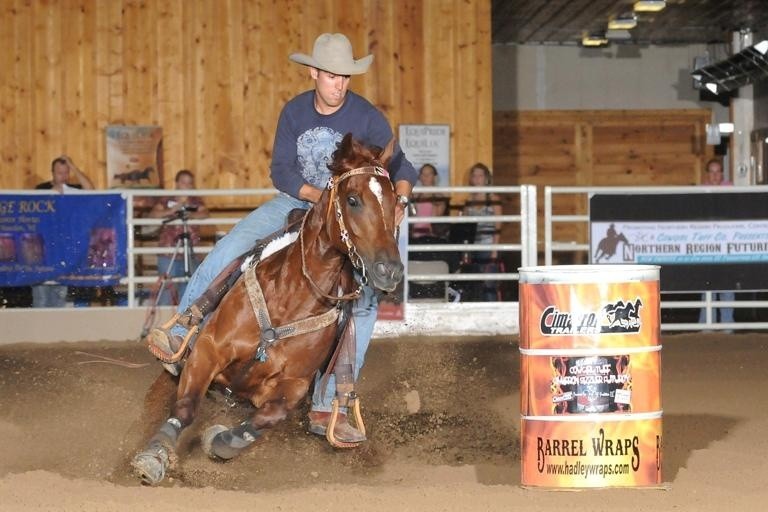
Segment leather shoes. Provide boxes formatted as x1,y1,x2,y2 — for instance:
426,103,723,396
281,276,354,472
152,327,189,377
309,411,366,443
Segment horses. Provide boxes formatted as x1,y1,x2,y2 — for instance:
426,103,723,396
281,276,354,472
129,129,406,491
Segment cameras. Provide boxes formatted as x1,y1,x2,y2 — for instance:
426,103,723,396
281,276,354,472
181,202,198,212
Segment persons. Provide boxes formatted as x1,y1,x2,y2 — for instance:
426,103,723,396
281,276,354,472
133,168,215,305
150,32,422,443
697,159,740,336
406,161,449,239
30,155,96,308
405,215,459,300
459,160,506,301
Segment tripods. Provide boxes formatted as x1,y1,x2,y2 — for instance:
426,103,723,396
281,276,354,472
141,232,195,340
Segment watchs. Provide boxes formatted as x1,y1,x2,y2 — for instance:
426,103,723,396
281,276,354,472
395,193,409,209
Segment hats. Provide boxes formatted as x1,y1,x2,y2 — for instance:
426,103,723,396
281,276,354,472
289,34,375,74
